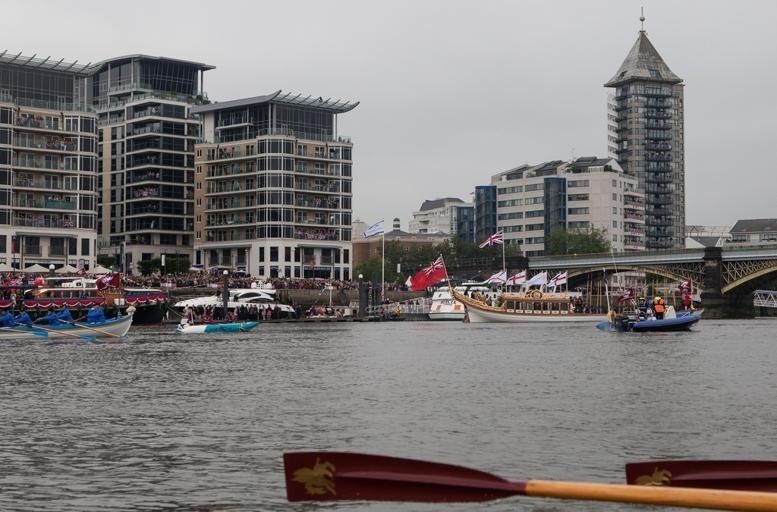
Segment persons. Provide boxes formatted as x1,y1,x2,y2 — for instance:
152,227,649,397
651,294,665,320
568,296,596,314
464,286,502,308
1,272,404,299
182,300,401,324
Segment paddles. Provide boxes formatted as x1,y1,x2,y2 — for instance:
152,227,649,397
624,461,777,494
282,451,777,512
13,322,96,341
0,328,49,337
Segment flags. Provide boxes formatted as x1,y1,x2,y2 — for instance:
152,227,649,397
410,257,448,292
479,231,503,249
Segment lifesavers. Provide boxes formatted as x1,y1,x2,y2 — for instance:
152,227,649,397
532,289,543,299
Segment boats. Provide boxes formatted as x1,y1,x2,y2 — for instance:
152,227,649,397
4,288,135,340
176,309,260,336
595,249,710,336
447,229,650,325
427,282,494,322
